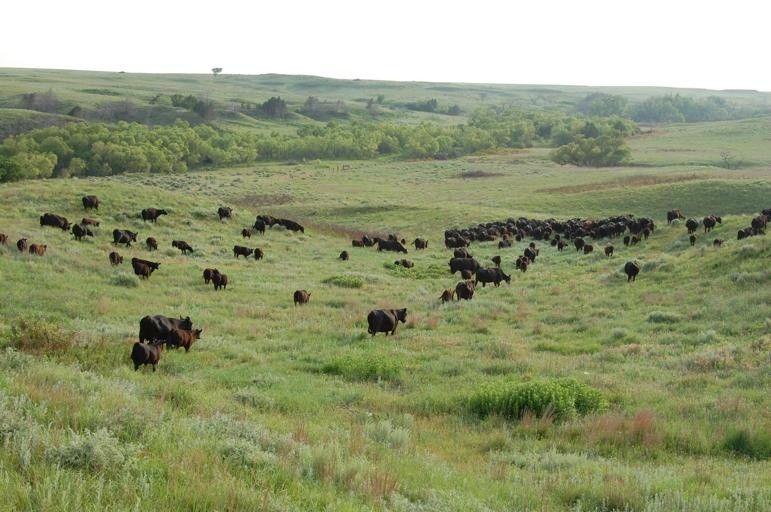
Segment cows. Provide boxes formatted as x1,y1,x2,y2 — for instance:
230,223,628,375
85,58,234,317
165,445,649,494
440,289,454,303
0,231,47,257
666,209,724,247
40,196,195,281
241,214,306,239
442,214,655,283
350,231,429,255
394,258,414,269
217,206,233,220
340,251,349,261
130,314,202,373
367,307,407,337
231,245,264,260
294,289,311,306
202,267,229,291
454,280,476,300
736,209,771,240
